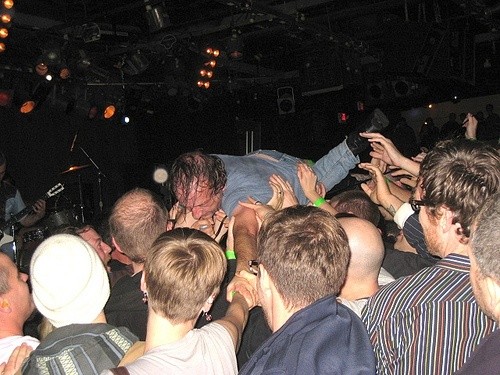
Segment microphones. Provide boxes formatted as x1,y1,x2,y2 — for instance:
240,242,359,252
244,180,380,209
70,132,78,152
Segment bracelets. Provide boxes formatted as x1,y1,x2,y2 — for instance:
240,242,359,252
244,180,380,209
225,249,236,260
313,197,324,207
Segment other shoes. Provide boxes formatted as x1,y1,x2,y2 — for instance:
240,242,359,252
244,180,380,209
347,110,390,153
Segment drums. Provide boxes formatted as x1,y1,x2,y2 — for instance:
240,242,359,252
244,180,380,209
24,225,51,245
43,206,78,231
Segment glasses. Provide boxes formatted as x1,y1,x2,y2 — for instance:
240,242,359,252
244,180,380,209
408,196,443,211
247,259,263,275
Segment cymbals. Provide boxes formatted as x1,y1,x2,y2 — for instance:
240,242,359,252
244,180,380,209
58,163,93,175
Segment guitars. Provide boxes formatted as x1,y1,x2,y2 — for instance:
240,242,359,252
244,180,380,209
0,182,65,247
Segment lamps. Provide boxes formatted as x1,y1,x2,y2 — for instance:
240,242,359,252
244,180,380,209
0,0,500,122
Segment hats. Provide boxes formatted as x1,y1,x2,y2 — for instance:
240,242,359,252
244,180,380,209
27,233,111,327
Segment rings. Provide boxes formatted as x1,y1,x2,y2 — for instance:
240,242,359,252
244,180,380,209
199,225,207,229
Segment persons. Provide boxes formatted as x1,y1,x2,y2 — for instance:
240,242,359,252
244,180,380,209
0,110,500,374
